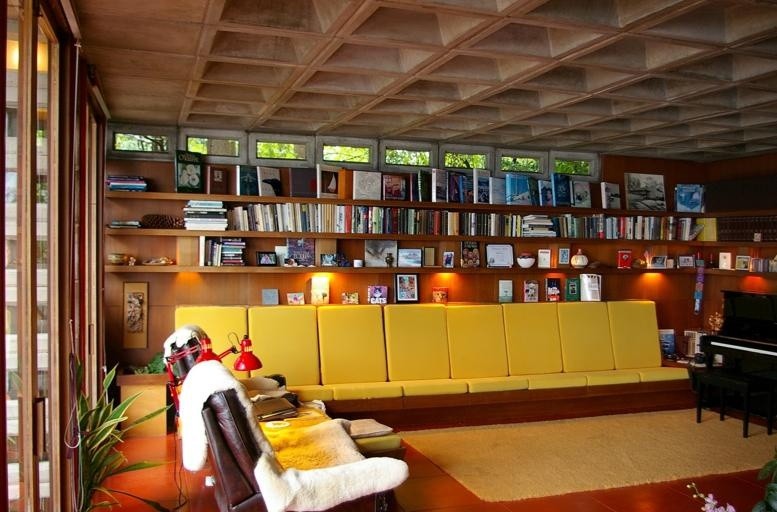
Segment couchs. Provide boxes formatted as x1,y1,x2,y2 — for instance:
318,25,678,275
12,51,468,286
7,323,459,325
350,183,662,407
158,300,696,432
164,321,406,512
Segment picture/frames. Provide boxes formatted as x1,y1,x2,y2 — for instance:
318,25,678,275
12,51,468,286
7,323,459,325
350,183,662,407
121,282,149,349
316,163,341,199
677,254,695,269
394,273,420,303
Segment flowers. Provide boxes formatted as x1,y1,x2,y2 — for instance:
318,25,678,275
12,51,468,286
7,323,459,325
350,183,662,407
687,482,737,512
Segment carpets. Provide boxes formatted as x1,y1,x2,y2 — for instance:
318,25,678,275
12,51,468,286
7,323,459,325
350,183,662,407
393,404,776,502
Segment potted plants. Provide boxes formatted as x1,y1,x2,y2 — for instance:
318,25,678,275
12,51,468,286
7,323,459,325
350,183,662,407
115,352,170,436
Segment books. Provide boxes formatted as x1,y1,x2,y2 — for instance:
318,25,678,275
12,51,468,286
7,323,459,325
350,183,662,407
107,220,142,229
262,277,387,304
275,238,337,267
289,164,591,208
254,396,298,422
433,287,449,304
183,200,717,241
175,151,282,197
718,251,777,272
499,274,602,302
365,239,480,269
105,175,148,192
601,173,705,213
176,235,246,266
717,216,777,242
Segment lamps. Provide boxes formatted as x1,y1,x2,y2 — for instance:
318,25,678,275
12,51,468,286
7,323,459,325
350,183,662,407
165,333,262,443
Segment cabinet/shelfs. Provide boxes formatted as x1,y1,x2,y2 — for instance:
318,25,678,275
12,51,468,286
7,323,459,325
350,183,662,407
99,180,704,276
703,196,777,287
